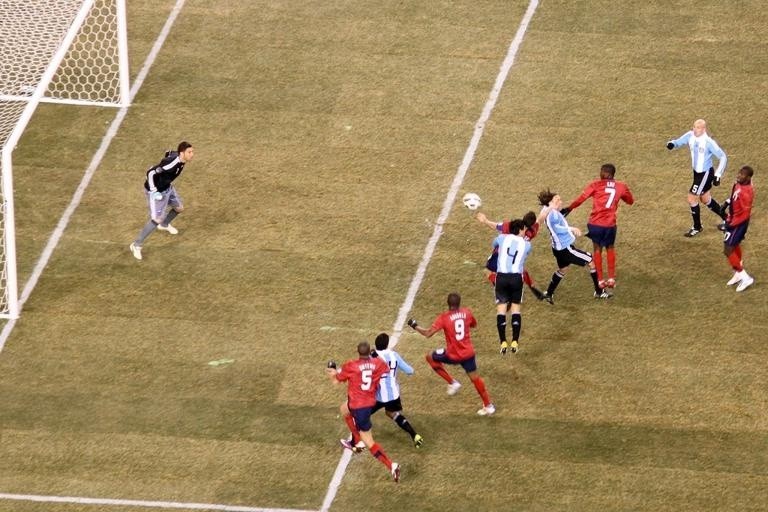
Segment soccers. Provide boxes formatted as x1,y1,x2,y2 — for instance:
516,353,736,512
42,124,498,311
463,193,481,210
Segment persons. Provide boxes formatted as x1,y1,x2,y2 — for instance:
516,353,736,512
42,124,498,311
493,219,533,354
560,163,636,290
476,201,558,303
127,140,195,260
665,119,731,236
326,341,401,482
719,166,755,291
340,333,423,454
406,293,496,416
536,187,613,304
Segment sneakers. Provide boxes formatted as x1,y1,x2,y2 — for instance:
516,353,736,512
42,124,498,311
157,224,178,235
339,428,425,482
498,340,522,357
685,222,724,237
727,269,754,292
542,279,616,304
447,379,495,417
130,243,142,259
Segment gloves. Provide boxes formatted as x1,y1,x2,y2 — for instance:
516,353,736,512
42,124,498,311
560,207,570,218
407,318,418,329
667,143,722,188
327,345,381,368
